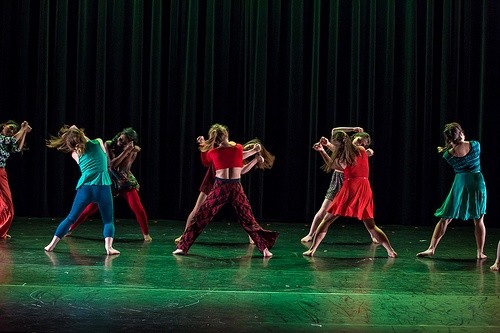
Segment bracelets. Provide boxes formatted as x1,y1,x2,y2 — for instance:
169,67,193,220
254,147,257,153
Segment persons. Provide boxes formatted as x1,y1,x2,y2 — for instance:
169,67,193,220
63,127,153,242
174,138,275,243
171,124,280,257
0,121,32,239
416,122,488,258
489,241,500,271
302,132,397,258
301,127,380,243
43,125,120,254
